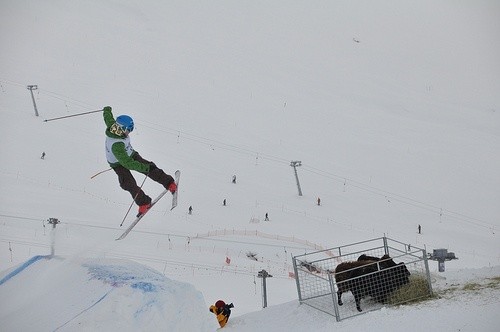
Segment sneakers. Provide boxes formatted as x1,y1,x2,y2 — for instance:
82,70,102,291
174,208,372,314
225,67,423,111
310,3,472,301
167,178,177,194
138,199,151,213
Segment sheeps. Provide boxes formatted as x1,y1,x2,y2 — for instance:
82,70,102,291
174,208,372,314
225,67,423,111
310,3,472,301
335,254,413,312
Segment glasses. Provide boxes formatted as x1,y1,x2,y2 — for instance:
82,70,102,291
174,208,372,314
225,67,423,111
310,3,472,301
128,126,133,131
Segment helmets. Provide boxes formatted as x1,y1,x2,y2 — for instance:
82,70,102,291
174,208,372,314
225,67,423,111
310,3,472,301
116,115,135,133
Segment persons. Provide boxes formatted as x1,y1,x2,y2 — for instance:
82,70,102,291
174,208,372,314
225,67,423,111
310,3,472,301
103,106,176,216
209,299,231,328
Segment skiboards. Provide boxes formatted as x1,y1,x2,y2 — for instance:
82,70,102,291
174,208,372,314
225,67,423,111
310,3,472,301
114,170,181,241
